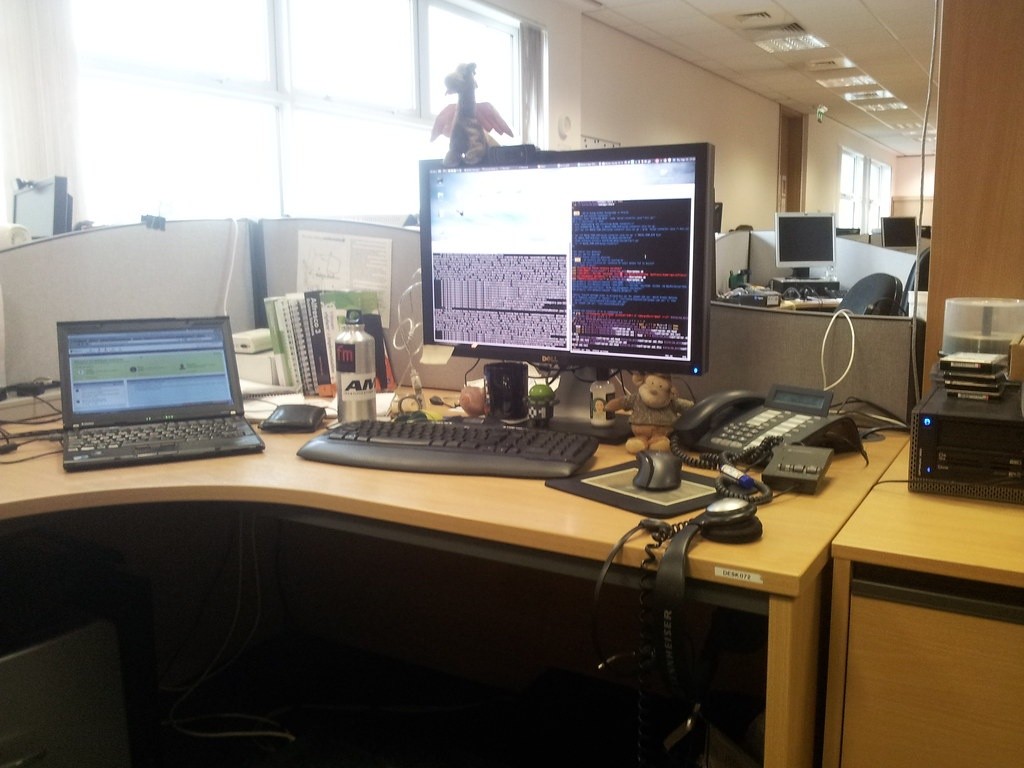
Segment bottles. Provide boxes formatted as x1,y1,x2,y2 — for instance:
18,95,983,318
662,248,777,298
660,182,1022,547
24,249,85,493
336,309,376,423
590,367,615,428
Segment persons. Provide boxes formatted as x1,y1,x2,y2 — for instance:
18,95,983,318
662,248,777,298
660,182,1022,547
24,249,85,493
729,225,753,231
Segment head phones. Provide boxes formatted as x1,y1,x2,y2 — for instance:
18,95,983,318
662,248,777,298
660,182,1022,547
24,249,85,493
651,496,763,707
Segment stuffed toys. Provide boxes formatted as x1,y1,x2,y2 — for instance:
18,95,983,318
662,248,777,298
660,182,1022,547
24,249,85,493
604,370,695,455
430,61,514,166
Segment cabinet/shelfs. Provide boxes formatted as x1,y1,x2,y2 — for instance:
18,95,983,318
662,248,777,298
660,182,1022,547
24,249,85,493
825,437,1024,768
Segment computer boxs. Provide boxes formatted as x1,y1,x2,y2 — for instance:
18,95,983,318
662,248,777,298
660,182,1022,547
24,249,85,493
768,278,840,299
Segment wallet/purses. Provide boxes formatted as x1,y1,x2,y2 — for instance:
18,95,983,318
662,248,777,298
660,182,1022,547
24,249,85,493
258,404,326,434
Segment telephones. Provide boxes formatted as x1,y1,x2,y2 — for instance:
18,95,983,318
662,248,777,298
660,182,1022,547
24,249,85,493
675,385,862,467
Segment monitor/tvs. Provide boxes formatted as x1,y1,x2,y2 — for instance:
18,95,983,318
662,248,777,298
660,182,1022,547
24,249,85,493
13,175,73,240
419,143,717,445
881,217,918,254
775,213,836,280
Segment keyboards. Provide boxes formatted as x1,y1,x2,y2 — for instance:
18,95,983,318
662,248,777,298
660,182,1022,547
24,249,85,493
296,420,600,479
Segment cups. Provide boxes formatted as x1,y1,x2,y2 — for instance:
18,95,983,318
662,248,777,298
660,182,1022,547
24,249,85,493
826,270,837,281
484,363,529,422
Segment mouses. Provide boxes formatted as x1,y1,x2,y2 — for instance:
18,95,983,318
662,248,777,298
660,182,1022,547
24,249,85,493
633,449,683,490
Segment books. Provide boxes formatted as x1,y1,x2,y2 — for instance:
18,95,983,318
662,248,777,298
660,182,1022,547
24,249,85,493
264,288,396,395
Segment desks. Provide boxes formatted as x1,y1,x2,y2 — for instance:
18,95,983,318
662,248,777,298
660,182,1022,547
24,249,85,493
1,224,913,767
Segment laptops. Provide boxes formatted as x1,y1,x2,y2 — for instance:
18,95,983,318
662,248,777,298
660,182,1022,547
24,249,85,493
56,316,266,472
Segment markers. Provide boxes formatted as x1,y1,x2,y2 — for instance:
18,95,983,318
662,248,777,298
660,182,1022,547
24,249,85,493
722,464,754,487
